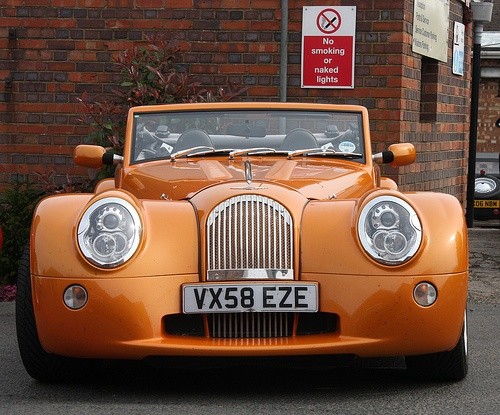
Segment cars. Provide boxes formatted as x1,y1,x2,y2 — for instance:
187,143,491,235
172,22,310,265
473,152,500,222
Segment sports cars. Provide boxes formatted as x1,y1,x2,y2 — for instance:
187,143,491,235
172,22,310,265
13,101,471,385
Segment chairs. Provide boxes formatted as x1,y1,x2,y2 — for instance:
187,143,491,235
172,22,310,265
173,128,212,155
281,128,319,150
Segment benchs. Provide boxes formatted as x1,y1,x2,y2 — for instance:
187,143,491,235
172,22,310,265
152,133,333,150
